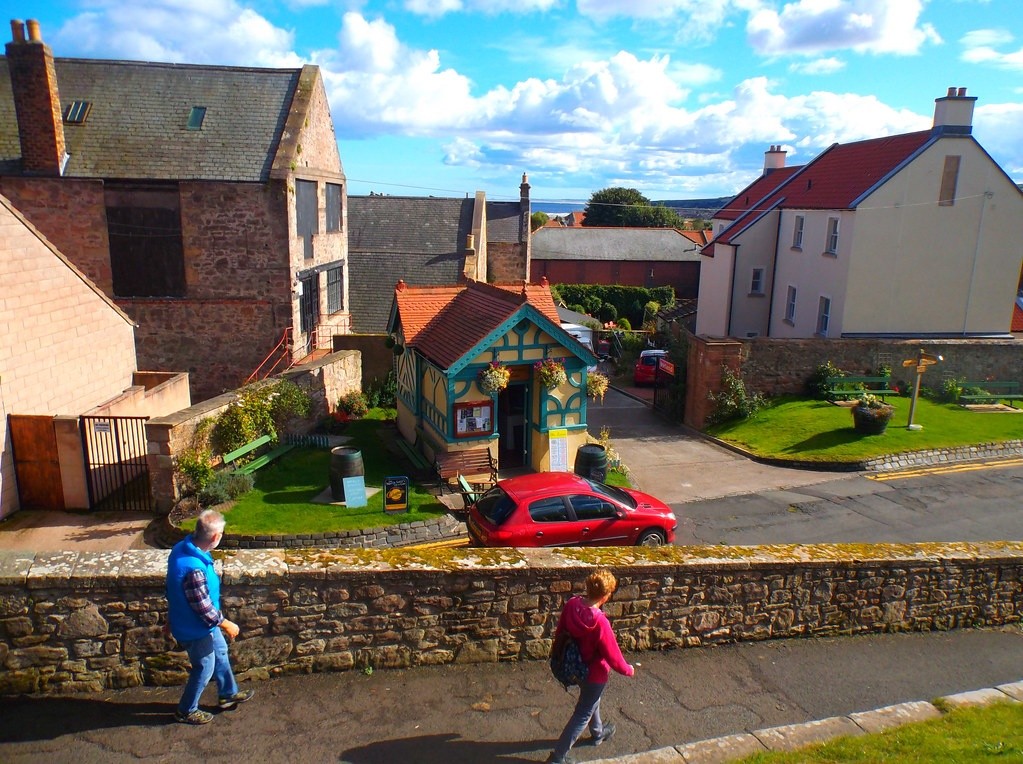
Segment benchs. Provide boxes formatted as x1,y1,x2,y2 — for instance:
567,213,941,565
955,381,1023,407
825,378,898,401
436,449,499,499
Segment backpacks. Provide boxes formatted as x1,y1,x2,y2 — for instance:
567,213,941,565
551,623,599,687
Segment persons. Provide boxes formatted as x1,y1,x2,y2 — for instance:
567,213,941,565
546,569,635,764
165,510,254,724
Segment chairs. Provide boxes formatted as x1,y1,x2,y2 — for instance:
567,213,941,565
456,469,495,520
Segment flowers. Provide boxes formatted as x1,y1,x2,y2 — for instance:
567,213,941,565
533,355,568,389
479,360,510,391
585,370,609,405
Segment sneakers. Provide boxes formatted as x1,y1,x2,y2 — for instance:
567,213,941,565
218,689,255,708
175,709,213,724
591,723,616,746
545,751,575,764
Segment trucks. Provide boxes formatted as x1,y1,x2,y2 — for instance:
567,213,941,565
561,322,595,354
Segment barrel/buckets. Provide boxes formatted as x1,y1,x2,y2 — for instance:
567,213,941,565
329,446,365,501
574,443,607,484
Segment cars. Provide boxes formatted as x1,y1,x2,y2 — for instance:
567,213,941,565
634,349,674,387
465,471,679,548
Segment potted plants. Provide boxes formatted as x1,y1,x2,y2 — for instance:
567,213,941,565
850,392,896,445
702,365,772,429
